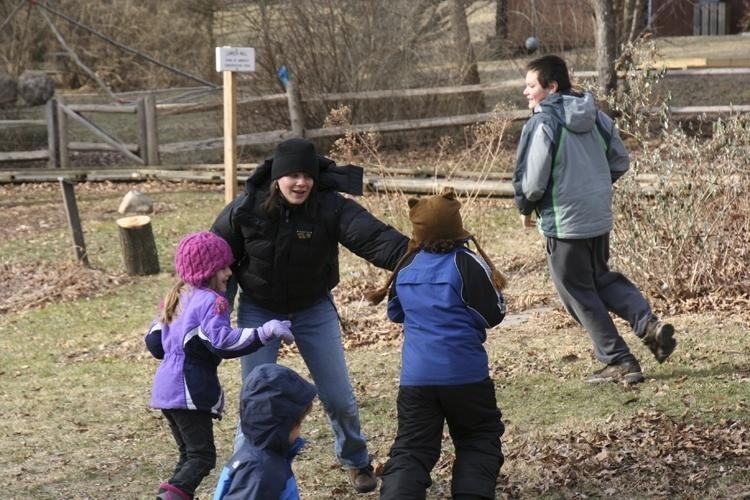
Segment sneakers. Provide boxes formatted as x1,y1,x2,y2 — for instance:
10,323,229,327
349,465,377,493
650,323,677,364
584,359,644,384
156,482,193,500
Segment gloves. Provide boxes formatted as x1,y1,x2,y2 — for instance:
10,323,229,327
256,320,295,345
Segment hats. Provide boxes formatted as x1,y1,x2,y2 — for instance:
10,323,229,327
159,232,233,314
271,139,319,182
368,187,507,305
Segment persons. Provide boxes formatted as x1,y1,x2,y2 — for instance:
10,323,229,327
145,230,294,499
511,54,677,385
211,362,319,499
207,136,412,494
368,190,508,500
